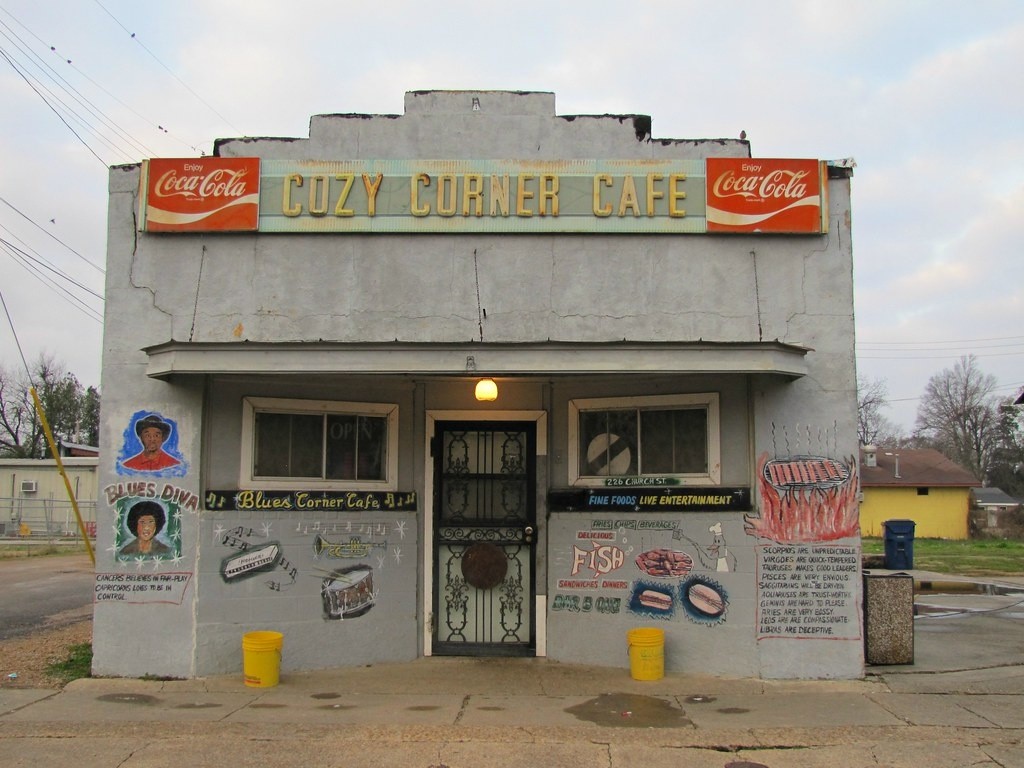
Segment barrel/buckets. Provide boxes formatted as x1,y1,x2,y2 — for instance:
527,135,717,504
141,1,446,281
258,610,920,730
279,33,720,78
242,631,284,688
626,627,665,680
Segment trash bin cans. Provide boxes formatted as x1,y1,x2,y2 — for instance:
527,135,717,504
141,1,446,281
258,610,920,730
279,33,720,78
881,518,917,571
862,570,914,666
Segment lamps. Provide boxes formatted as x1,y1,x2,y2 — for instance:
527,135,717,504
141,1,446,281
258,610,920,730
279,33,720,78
474,378,498,402
471,96,481,112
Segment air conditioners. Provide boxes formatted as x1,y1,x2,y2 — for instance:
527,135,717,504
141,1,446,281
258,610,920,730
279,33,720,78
20,480,36,492
